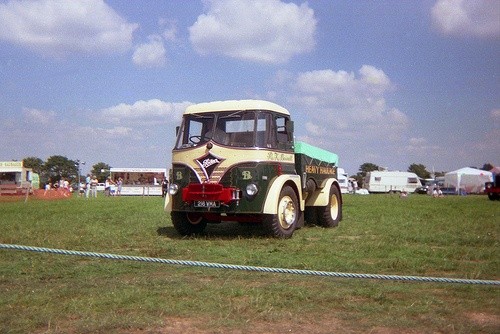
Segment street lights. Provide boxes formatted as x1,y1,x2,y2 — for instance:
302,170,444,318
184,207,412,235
74,159,86,192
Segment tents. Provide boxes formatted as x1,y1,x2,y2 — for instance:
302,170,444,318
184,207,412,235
444,166,494,195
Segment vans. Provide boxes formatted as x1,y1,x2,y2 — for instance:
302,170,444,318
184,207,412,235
416,180,456,194
364,170,422,193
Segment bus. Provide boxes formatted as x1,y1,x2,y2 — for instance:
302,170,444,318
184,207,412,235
163,99,343,239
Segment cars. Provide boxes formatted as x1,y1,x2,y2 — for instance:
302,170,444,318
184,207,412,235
80,182,86,188
96,183,105,190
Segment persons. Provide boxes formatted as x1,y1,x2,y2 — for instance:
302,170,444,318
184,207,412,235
161,177,168,197
59,177,69,189
348,180,358,194
84,174,98,198
117,176,123,195
105,177,113,190
